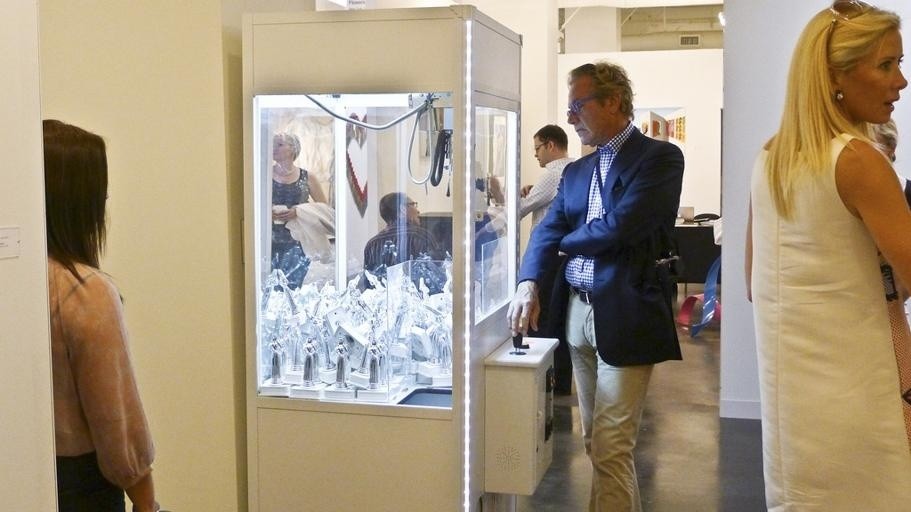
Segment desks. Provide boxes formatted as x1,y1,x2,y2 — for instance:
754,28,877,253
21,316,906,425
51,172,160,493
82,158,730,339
671,218,722,295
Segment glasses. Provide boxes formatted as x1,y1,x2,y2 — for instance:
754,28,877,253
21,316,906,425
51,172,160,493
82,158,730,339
407,202,417,207
535,142,550,154
567,95,599,118
828,0,871,38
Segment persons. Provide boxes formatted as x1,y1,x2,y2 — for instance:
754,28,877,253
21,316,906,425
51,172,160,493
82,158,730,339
508,63,685,510
859,110,911,300
363,190,446,292
272,133,325,286
490,124,582,394
42,116,164,512
746,3,911,510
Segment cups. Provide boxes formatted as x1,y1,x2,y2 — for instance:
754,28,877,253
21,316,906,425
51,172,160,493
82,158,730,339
272,206,288,225
682,206,695,222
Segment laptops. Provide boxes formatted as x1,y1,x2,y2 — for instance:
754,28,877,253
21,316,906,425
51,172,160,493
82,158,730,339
677,206,694,222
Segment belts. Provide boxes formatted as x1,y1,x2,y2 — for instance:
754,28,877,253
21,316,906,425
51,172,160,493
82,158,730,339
575,288,592,306
